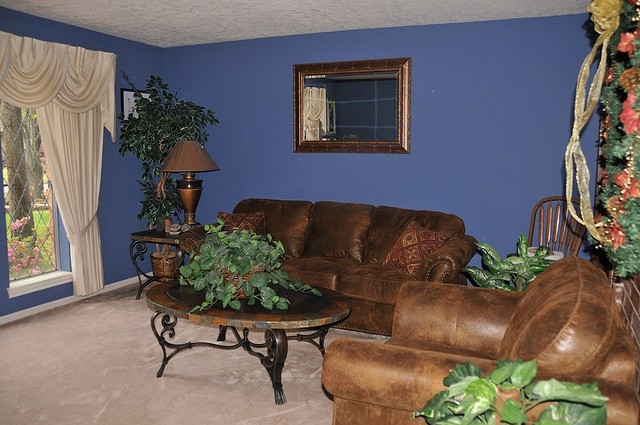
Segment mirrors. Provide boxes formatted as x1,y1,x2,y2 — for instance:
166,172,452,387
293,56,412,154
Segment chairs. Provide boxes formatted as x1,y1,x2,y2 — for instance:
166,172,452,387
523,195,586,256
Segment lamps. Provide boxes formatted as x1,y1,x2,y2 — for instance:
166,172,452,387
160,140,220,228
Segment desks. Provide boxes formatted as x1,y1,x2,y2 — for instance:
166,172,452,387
129,229,186,300
145,280,352,405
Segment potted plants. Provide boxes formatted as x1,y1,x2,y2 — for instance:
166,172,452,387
116,69,220,283
178,216,322,313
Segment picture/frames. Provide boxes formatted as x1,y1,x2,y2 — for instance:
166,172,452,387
325,99,337,136
120,87,152,124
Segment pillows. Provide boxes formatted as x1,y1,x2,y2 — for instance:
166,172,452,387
382,219,452,277
256,214,311,259
217,211,265,235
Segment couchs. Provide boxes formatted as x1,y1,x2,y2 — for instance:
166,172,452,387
321,254,640,425
179,198,478,336
